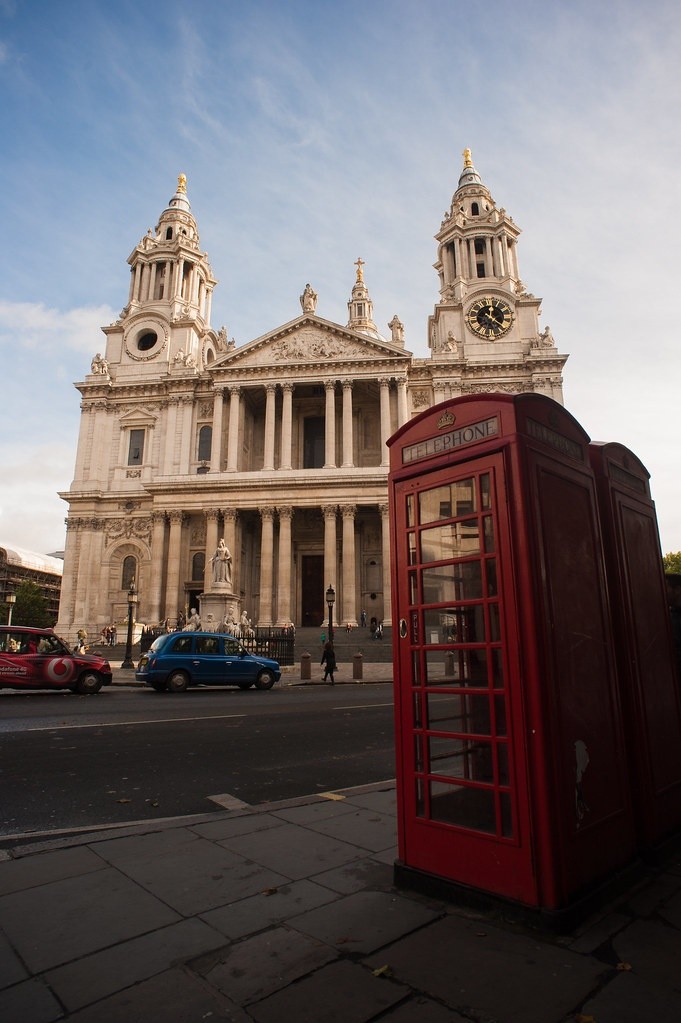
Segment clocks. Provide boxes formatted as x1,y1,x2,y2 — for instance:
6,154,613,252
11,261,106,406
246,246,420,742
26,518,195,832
464,296,515,341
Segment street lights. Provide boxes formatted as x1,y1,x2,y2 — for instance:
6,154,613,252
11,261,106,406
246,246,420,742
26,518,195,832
5,587,18,652
323,584,339,672
120,586,138,669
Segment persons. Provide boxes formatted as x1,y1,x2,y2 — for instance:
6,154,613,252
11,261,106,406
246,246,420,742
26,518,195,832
320,642,335,686
347,609,384,641
388,314,404,342
444,637,457,673
321,631,326,645
302,284,317,311
208,538,232,583
180,302,191,320
223,606,243,637
182,608,201,632
100,624,117,647
442,330,457,353
282,623,295,647
174,348,191,366
240,610,256,643
161,611,183,632
218,326,228,345
537,326,555,347
90,353,109,374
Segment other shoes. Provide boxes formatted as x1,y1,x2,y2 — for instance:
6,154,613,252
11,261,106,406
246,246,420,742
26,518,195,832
330,682,334,685
322,678,326,681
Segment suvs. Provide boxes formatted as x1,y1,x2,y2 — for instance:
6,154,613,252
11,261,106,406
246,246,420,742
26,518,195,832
134,630,282,694
0,623,114,695
